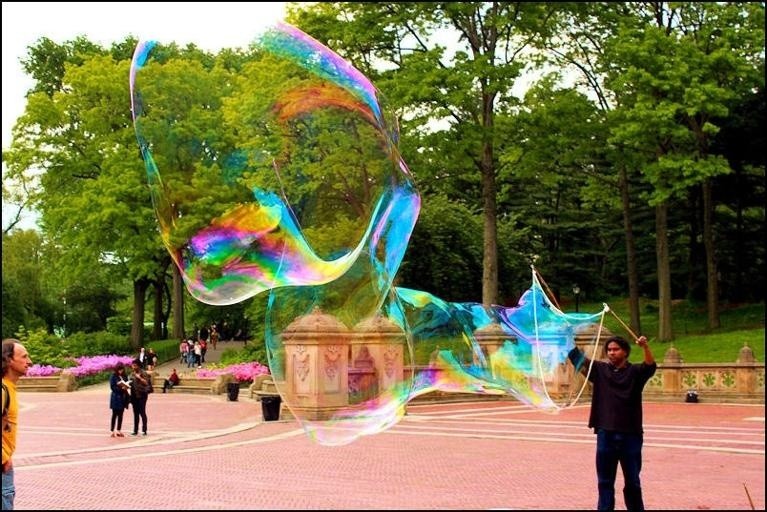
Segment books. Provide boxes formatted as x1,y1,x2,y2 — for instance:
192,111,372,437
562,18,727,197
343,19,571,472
116,379,131,391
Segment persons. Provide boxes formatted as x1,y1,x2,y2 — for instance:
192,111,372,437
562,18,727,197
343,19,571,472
110,361,130,437
192,322,198,342
199,324,209,345
160,368,178,394
210,323,221,350
147,348,159,372
197,336,209,362
130,359,149,435
566,323,657,511
179,339,189,364
138,348,148,370
0,339,32,510
193,342,203,368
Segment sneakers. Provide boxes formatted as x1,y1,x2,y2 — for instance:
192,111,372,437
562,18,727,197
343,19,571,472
131,432,137,436
110,433,118,438
117,432,125,438
140,431,147,437
